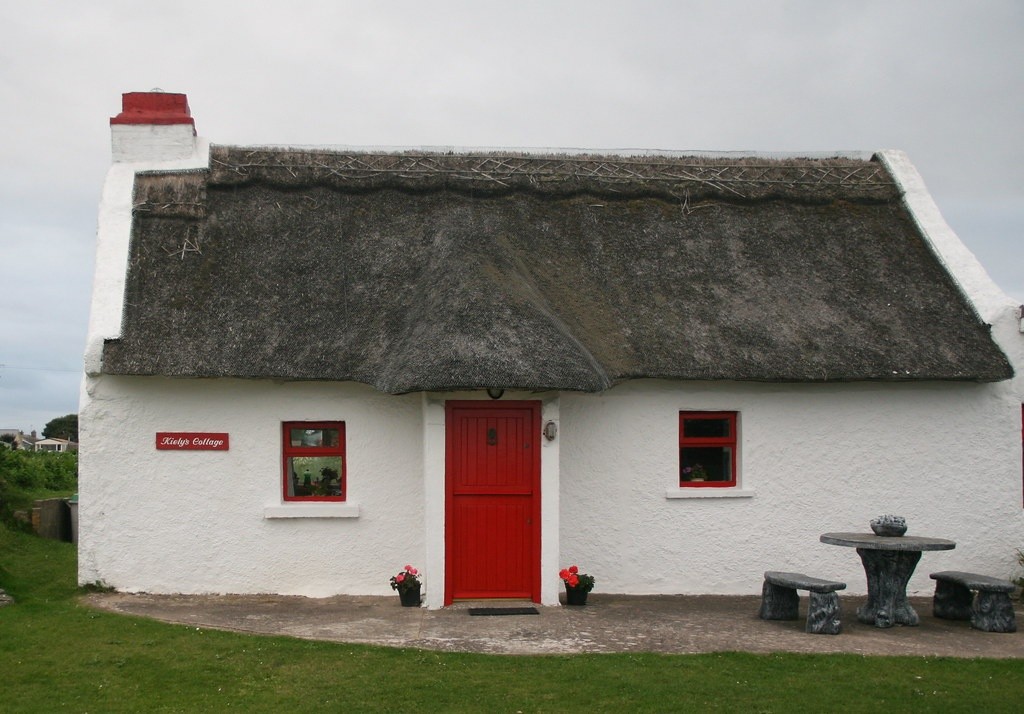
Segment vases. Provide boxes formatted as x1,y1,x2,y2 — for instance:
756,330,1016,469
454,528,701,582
566,587,588,606
691,478,704,481
399,581,420,607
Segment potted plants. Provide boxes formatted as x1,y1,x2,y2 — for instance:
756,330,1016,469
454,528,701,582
871,514,907,537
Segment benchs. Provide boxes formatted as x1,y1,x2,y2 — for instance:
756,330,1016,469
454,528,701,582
758,571,846,635
930,571,1017,633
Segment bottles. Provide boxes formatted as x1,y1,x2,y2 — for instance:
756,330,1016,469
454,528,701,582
304,469,311,488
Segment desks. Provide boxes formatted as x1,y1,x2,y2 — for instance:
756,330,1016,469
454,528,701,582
820,532,956,628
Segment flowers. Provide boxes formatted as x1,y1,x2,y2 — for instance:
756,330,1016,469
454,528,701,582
390,565,423,594
559,565,595,592
682,463,705,478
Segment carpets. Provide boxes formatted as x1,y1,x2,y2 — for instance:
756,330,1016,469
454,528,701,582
468,608,540,616
